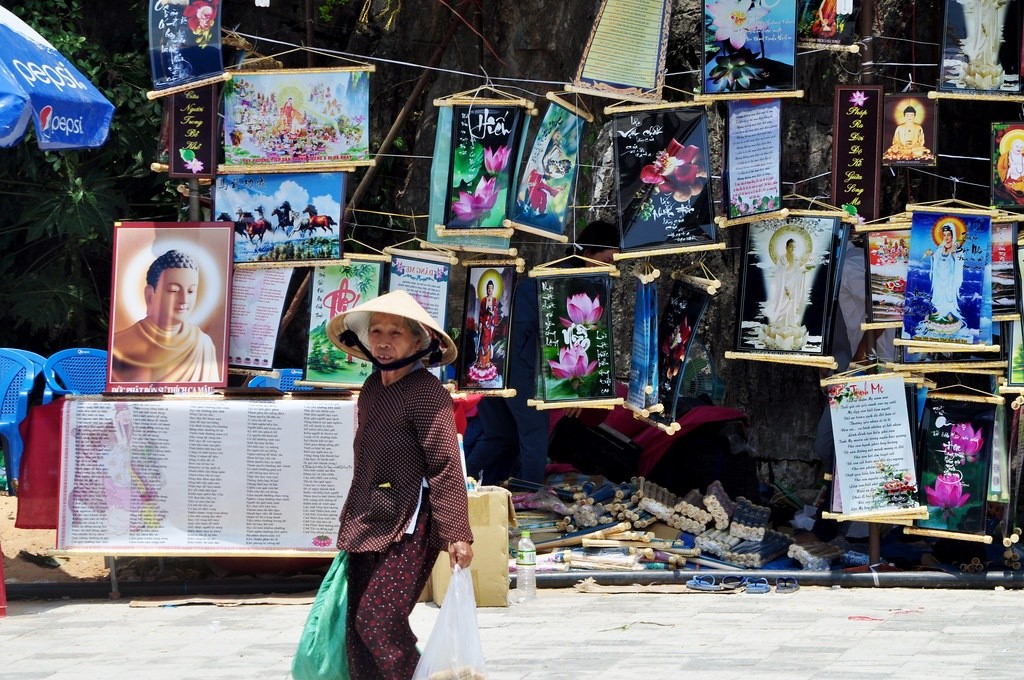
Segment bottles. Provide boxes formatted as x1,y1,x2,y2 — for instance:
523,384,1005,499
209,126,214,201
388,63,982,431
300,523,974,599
516,532,537,603
841,550,895,567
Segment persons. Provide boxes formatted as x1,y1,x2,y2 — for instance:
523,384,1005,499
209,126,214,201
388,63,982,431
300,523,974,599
327,290,473,680
814,237,926,545
462,219,623,491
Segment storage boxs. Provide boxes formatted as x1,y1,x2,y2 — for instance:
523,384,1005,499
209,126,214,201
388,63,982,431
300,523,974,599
429,485,519,608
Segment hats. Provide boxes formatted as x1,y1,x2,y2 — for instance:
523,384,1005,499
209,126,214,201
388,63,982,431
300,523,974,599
327,290,457,368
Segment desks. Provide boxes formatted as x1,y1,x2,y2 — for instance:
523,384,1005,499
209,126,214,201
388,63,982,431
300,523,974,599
57,386,467,600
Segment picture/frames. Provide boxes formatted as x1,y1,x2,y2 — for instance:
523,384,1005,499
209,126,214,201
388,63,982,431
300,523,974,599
106,221,235,394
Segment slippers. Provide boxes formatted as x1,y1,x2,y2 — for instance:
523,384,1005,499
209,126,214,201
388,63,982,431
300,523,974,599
775,576,800,594
720,576,747,589
745,578,772,593
686,576,723,590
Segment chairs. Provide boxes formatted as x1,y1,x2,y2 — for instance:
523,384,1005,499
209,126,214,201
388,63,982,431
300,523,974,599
0,348,110,499
248,368,346,392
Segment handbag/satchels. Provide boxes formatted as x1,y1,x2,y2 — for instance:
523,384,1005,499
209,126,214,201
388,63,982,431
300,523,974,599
290,549,351,680
411,562,491,680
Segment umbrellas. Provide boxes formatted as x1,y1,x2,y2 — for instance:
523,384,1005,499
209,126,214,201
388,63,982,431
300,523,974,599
0,4,117,152
